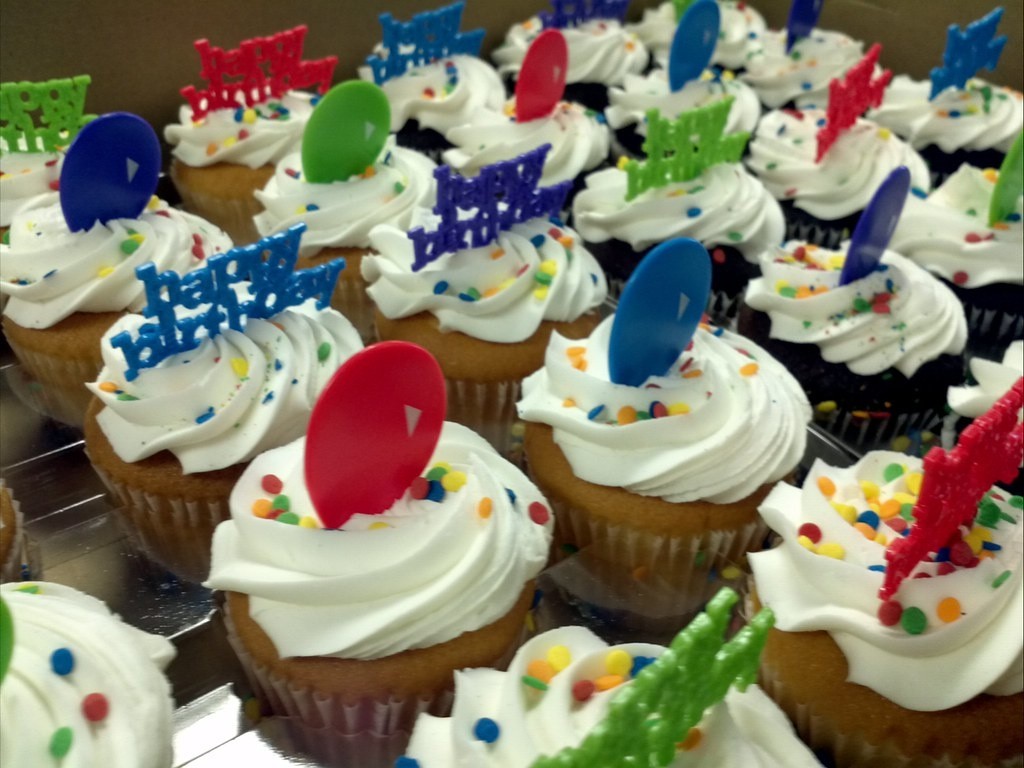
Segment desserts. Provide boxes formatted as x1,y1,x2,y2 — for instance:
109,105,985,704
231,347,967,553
0,0,1024,768
